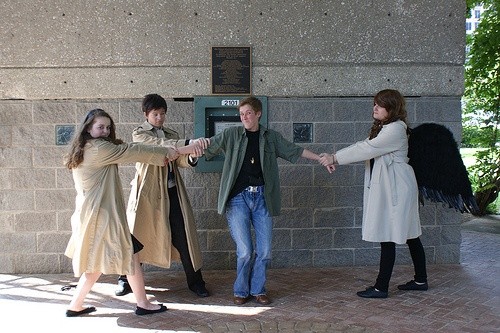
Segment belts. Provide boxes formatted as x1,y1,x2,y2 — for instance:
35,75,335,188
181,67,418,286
244,186,260,192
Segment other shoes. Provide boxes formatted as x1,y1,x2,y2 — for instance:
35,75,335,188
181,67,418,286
234,297,247,304
255,295,270,305
135,304,167,315
115,284,131,296
188,286,209,297
357,286,388,298
66,306,95,317
397,280,428,291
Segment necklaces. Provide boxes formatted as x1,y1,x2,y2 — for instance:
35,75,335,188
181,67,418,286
248,147,258,164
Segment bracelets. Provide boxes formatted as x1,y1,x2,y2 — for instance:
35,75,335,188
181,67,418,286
332,154,336,165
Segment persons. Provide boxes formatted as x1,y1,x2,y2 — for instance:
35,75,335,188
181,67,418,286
190,96,335,305
116,93,210,297
64,109,202,317
318,89,428,298
